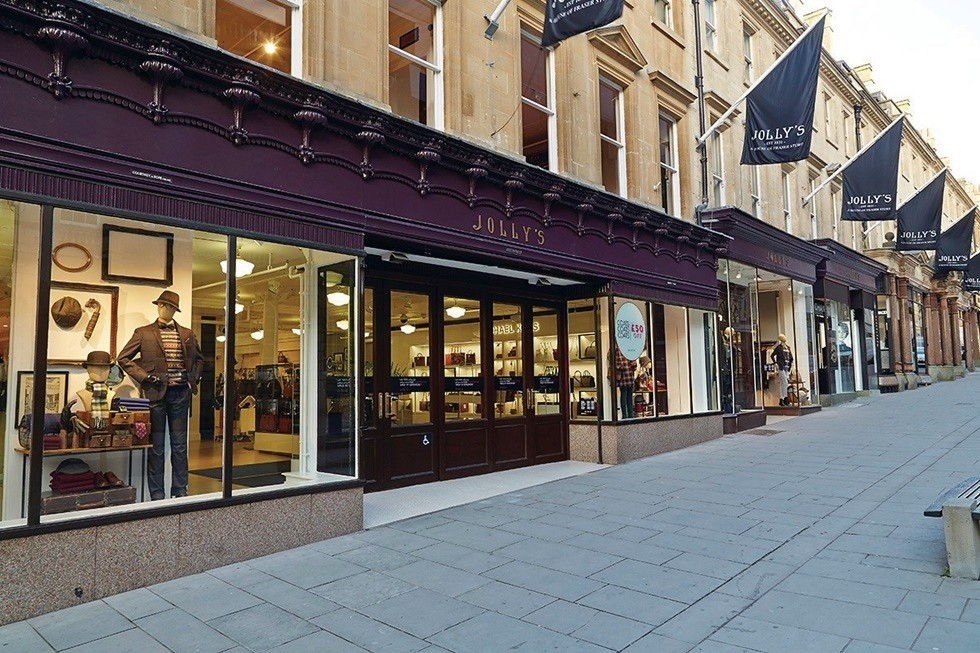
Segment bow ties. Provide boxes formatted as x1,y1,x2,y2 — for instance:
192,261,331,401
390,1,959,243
158,323,175,330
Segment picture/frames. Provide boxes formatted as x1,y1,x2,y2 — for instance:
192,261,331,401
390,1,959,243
15,371,69,429
101,223,173,286
47,282,119,365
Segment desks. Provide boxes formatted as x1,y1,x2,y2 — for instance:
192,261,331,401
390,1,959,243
14,445,154,518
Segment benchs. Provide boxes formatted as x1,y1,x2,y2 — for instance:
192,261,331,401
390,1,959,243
923,477,980,580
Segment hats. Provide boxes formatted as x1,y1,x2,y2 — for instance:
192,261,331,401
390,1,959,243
55,457,90,474
82,351,114,368
639,356,651,367
152,291,181,312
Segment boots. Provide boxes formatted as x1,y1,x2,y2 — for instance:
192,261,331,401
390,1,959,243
784,397,790,406
779,398,786,406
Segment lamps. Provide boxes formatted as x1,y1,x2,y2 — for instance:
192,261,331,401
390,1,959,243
446,299,466,318
336,315,348,330
220,252,255,278
400,319,416,334
223,299,244,314
327,286,350,306
250,329,264,340
291,325,304,335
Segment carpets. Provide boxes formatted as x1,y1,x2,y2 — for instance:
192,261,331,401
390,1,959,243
189,460,291,488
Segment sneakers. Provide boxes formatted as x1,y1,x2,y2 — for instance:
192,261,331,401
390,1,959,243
92,470,109,487
104,471,122,487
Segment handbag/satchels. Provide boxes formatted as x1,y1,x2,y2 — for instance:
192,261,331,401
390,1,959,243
496,347,516,358
535,342,558,361
510,409,518,414
633,371,653,416
537,366,559,385
445,346,476,365
585,340,597,358
445,392,480,412
419,393,431,411
581,397,598,414
497,402,504,413
573,370,595,387
414,353,426,366
496,369,503,376
509,361,515,377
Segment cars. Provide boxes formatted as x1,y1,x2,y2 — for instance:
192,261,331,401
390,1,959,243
823,338,875,369
915,337,926,365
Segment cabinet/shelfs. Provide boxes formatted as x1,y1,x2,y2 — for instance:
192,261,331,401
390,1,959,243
410,333,668,418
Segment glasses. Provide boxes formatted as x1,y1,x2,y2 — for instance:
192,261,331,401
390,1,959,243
158,302,174,308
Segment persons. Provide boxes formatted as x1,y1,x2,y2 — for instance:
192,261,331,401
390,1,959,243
118,291,204,502
639,356,652,369
771,334,793,406
77,351,116,411
723,327,736,402
838,322,851,351
616,347,637,419
